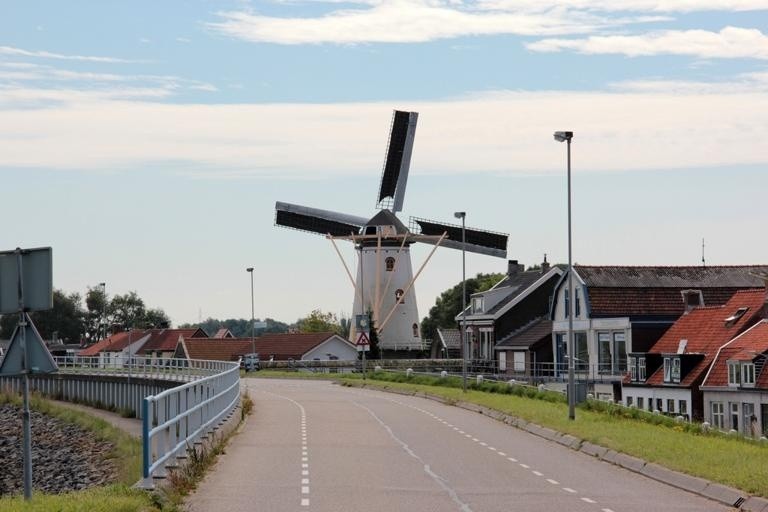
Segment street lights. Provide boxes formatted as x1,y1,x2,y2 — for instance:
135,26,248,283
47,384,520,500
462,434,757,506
100,282,107,356
453,210,468,393
245,266,256,352
553,129,578,419
